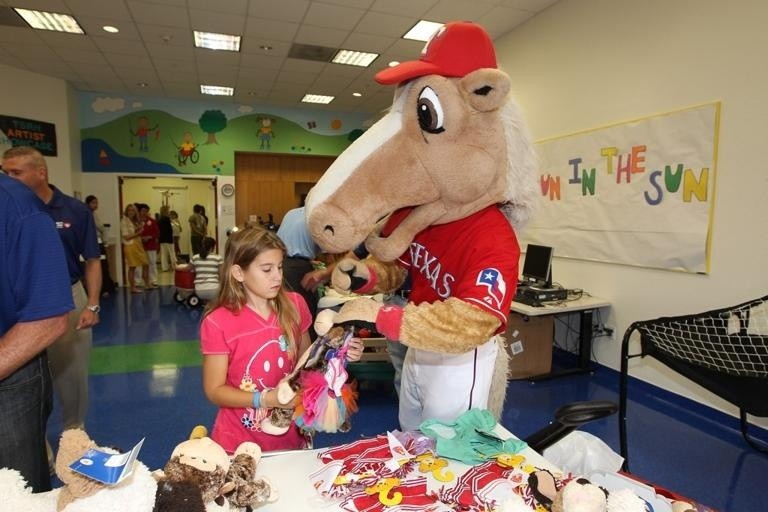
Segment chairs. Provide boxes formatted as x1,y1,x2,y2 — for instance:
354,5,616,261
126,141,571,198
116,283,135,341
620,295,768,474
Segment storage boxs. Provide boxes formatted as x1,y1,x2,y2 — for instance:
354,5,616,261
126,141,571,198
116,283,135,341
506,313,556,381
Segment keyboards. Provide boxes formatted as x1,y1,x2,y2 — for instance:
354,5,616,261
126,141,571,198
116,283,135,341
512,291,535,306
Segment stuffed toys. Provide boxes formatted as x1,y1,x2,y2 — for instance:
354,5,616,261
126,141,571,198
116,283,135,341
303,20,542,431
0,428,157,512
151,425,271,511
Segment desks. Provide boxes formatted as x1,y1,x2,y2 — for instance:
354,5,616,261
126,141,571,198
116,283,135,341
510,296,611,387
33,421,566,512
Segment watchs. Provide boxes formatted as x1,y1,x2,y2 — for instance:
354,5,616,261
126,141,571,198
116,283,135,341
86,304,100,312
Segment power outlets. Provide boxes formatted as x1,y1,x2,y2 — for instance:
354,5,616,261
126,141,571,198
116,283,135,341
605,325,616,340
593,323,603,336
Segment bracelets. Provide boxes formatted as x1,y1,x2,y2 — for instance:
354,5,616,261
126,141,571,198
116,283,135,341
253,391,260,407
260,387,276,409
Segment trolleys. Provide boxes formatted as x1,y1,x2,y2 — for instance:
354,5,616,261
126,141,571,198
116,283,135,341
172,262,202,307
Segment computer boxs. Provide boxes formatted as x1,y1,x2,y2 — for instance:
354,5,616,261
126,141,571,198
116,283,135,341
523,289,568,301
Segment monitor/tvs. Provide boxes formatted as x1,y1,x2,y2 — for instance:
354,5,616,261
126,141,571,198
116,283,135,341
521,243,560,291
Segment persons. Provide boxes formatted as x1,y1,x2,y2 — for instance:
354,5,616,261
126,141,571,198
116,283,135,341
276,206,320,342
188,237,224,299
200,225,363,454
188,204,208,255
2,173,78,494
86,195,181,294
1,148,103,474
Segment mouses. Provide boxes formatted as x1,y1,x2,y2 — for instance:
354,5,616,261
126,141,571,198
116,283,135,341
531,301,543,307
